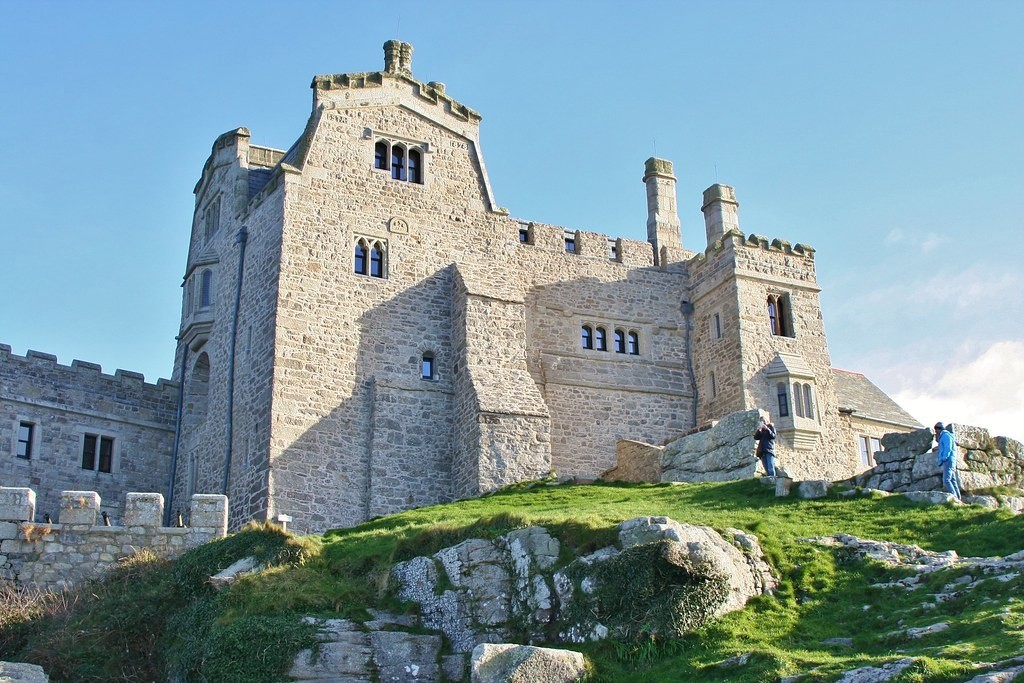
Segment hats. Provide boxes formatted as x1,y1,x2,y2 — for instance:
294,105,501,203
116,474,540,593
934,422,944,430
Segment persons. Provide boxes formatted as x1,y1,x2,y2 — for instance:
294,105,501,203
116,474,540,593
754,416,777,476
934,422,961,500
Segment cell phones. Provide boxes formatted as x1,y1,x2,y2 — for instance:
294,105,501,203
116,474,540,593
759,421,764,426
